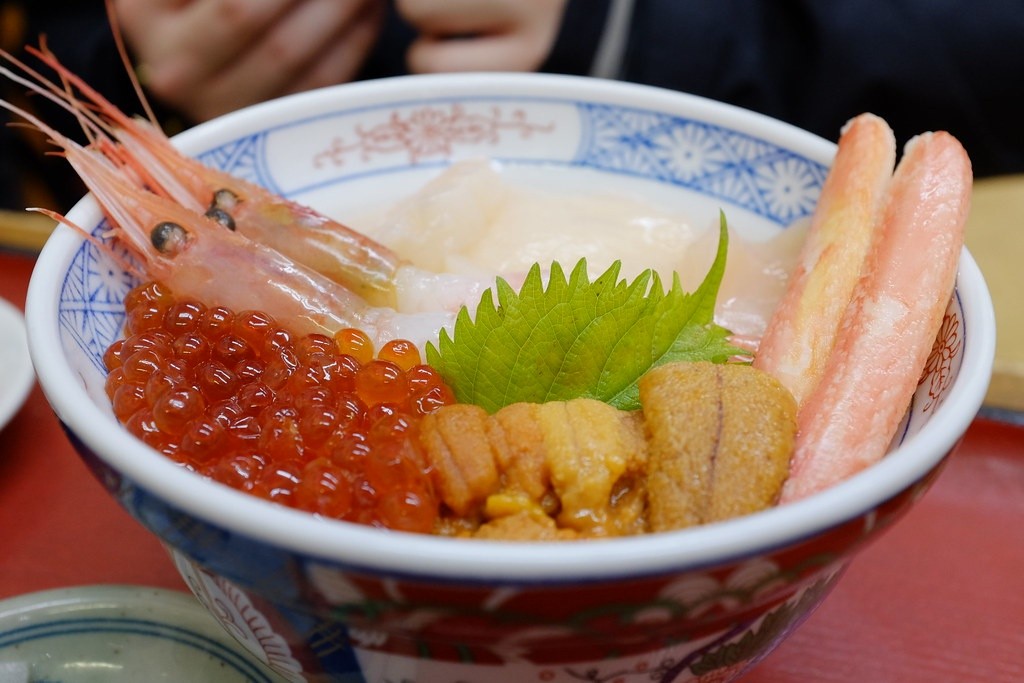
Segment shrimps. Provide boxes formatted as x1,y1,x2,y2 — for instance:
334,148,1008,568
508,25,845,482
0,0,806,365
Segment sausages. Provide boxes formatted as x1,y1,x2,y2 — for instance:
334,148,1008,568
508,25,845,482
754,114,973,508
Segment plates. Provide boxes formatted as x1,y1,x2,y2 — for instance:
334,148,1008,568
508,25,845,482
0,583,277,683
0,293,36,428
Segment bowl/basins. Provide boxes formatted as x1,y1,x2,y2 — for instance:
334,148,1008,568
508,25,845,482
28,72,994,683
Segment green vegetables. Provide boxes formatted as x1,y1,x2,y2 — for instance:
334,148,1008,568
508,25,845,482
424,207,756,408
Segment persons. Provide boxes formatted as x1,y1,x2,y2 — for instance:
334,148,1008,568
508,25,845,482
0,0,1024,183
